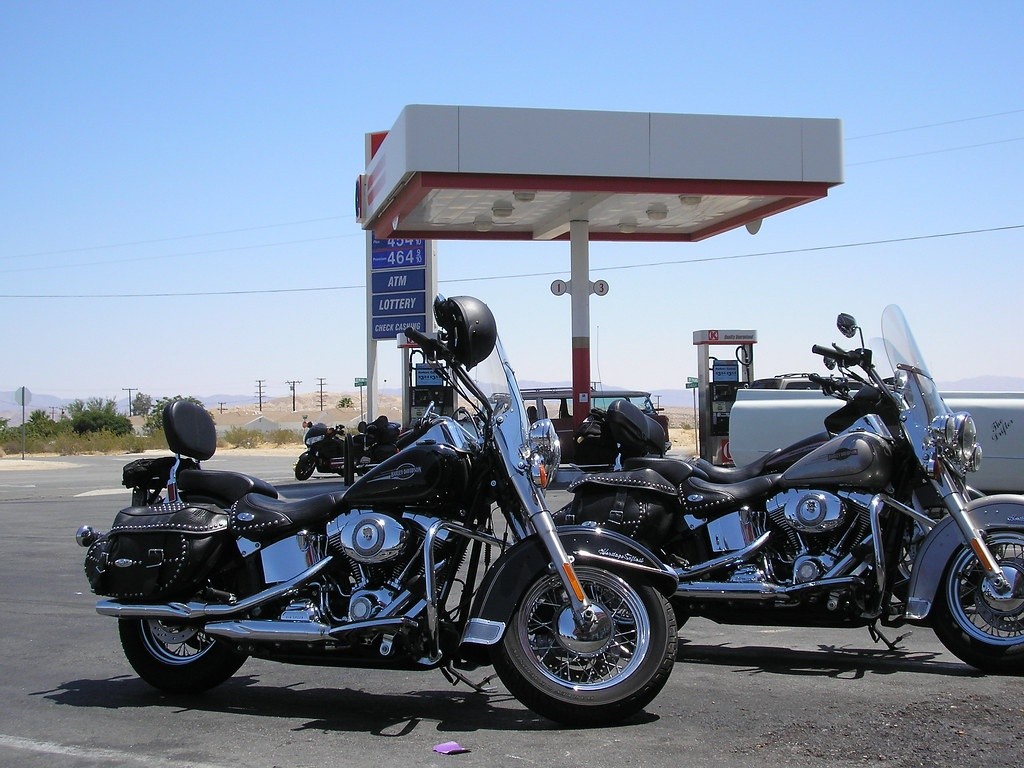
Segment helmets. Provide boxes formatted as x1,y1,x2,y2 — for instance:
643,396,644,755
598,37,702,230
824,382,906,448
440,296,497,372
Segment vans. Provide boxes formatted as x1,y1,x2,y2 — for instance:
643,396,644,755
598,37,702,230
480,387,672,466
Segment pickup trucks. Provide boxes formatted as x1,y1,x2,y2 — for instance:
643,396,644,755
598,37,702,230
728,388,1024,612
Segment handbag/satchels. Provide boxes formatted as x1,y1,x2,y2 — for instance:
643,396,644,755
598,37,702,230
573,408,616,465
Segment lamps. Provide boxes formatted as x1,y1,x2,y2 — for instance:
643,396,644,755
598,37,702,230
473,215,494,232
618,221,641,233
646,209,670,221
492,202,515,217
678,194,702,206
515,192,536,202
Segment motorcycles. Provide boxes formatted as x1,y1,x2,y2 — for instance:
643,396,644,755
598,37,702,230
74,296,679,730
294,415,426,480
546,304,1024,676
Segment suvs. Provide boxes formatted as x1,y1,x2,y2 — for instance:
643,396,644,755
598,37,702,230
748,372,867,393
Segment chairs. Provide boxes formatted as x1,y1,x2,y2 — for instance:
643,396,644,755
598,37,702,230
526,405,537,418
